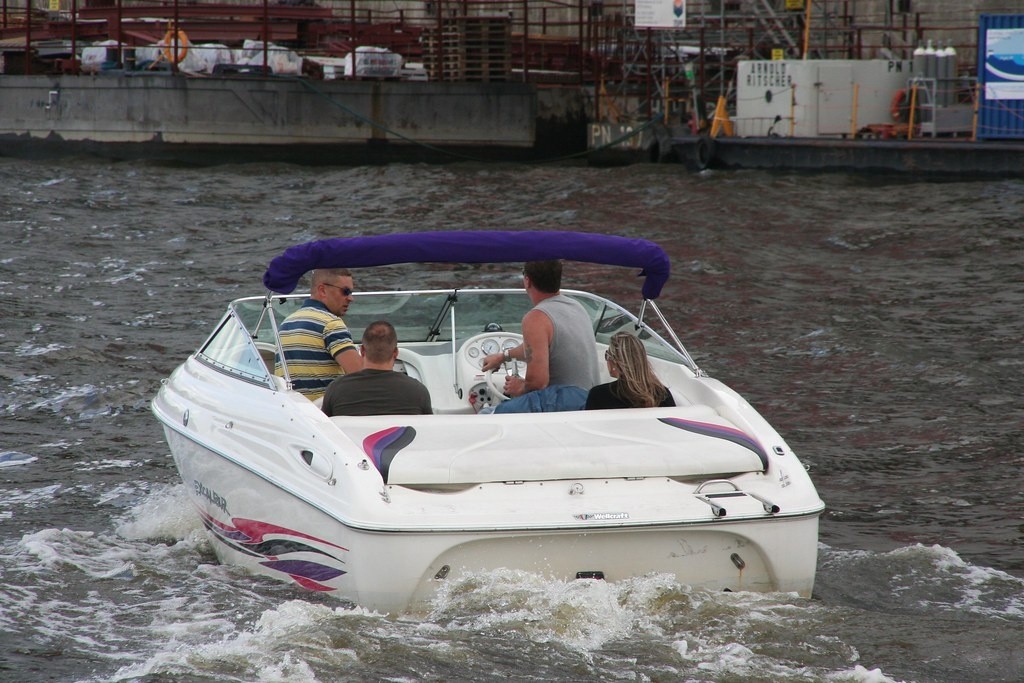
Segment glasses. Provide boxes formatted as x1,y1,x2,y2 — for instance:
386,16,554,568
320,282,353,297
603,349,615,365
521,268,526,277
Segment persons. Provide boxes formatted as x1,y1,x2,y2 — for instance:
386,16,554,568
481,259,601,399
274,269,364,403
585,330,677,410
320,319,434,416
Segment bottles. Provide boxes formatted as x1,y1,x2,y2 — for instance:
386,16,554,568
511,357,520,399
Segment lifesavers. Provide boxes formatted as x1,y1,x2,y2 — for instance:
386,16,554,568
893,88,907,119
166,27,187,62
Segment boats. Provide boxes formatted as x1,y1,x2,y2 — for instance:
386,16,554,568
149,230,829,623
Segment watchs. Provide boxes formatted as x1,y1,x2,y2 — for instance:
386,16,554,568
503,348,512,362
